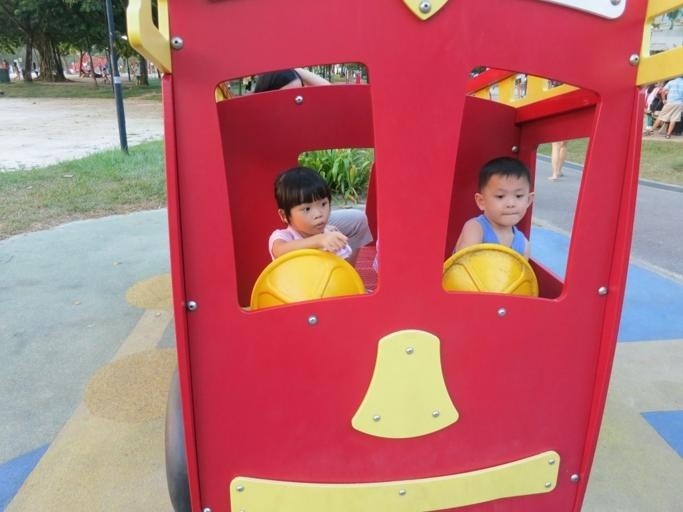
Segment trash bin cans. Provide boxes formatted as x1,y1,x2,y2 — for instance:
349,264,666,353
0,69,9,82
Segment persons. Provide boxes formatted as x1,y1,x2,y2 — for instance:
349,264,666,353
1,60,8,70
549,141,567,181
451,157,536,263
267,166,353,262
253,68,374,267
642,77,682,139
32,63,38,77
101,68,110,85
11,62,20,81
130,63,141,86
